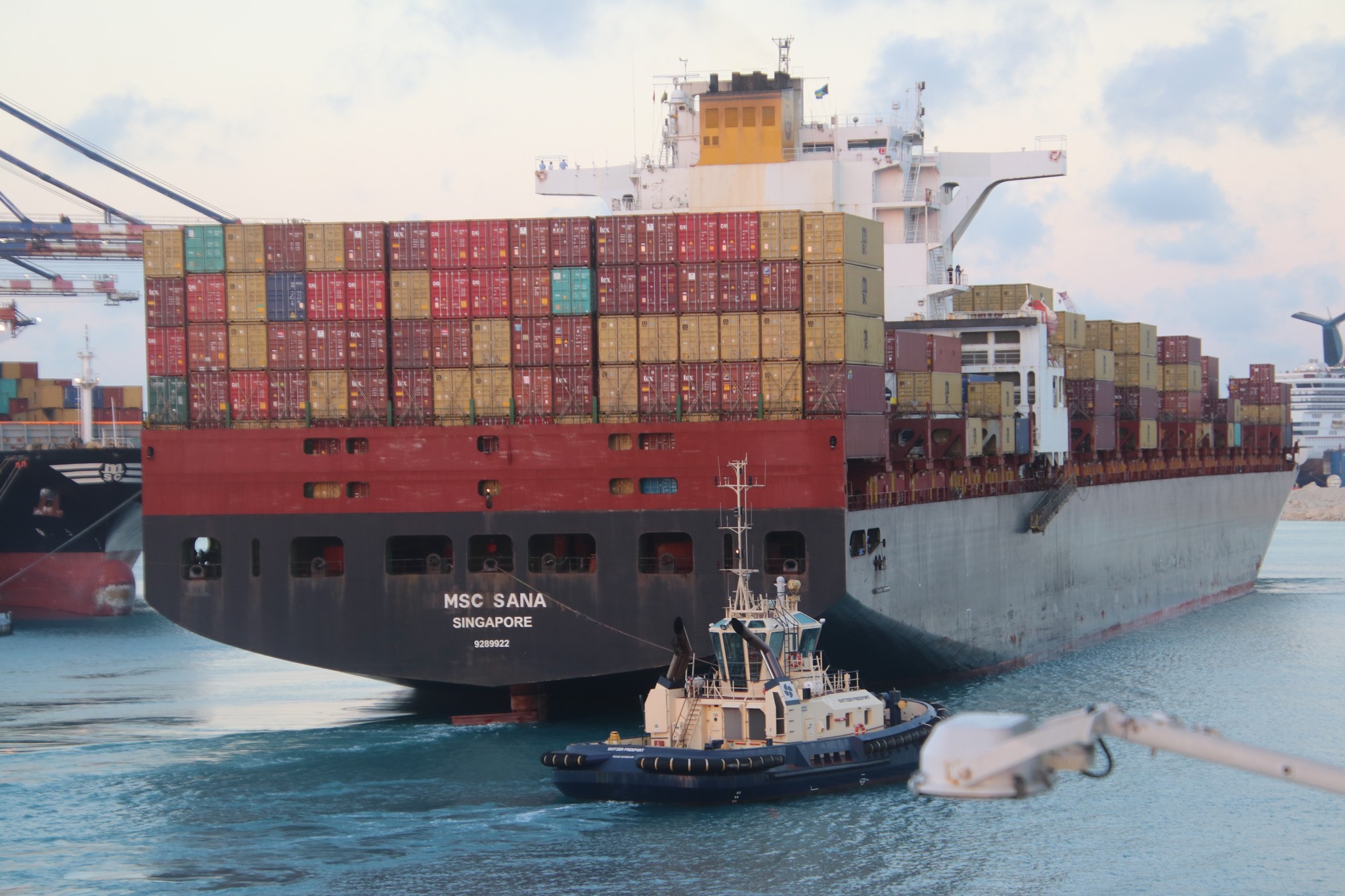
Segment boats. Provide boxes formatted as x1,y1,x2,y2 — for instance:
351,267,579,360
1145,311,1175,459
538,456,949,809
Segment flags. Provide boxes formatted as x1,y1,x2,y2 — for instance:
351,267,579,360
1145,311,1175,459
815,83,829,99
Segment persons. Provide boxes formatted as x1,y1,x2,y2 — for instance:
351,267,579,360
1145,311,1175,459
539,159,568,171
946,265,963,284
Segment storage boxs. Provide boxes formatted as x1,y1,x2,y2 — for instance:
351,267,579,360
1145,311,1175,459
0,212,1295,515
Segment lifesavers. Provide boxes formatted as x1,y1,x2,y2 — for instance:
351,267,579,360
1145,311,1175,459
788,651,801,667
687,676,693,690
538,171,546,180
855,724,865,736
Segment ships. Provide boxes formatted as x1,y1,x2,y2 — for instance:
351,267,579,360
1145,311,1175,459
1,100,245,624
1273,308,1345,488
137,28,1303,703
